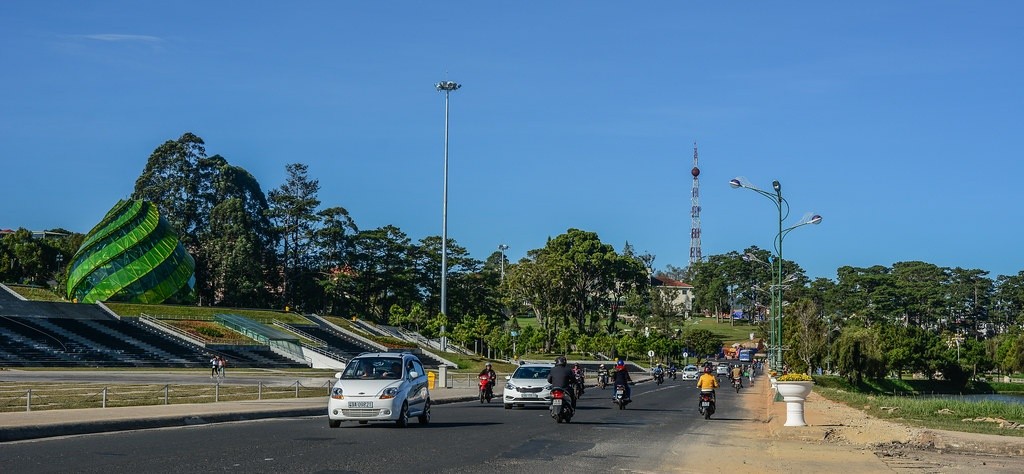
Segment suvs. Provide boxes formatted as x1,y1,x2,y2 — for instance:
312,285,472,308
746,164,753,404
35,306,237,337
328,352,431,428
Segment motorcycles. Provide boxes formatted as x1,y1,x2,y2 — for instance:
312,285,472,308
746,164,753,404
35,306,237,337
571,372,584,400
653,369,676,385
597,371,632,410
728,368,755,393
477,374,495,404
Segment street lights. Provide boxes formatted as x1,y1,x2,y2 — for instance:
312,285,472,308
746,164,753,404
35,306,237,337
729,179,825,376
497,245,509,282
435,80,463,351
740,253,800,372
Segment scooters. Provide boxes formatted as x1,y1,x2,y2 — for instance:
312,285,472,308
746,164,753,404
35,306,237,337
545,379,579,424
696,379,723,420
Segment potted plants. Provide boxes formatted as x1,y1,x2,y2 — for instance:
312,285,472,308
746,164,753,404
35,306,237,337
766,369,813,427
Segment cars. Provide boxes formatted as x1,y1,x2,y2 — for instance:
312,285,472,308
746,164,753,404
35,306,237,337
682,365,701,381
716,362,731,377
503,364,556,410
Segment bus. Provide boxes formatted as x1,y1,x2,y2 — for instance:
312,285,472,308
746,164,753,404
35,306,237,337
740,350,753,363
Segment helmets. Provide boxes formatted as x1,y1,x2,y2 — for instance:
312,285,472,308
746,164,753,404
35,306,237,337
734,364,739,368
703,366,710,372
617,360,624,366
485,362,492,367
555,357,567,366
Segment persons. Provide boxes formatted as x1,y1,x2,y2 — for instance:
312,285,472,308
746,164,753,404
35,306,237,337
597,363,610,384
546,357,580,413
572,362,586,394
477,363,497,397
727,363,757,389
668,361,677,380
695,360,721,407
611,359,633,403
285,304,291,312
382,361,401,380
362,364,380,376
652,363,663,381
210,354,226,379
